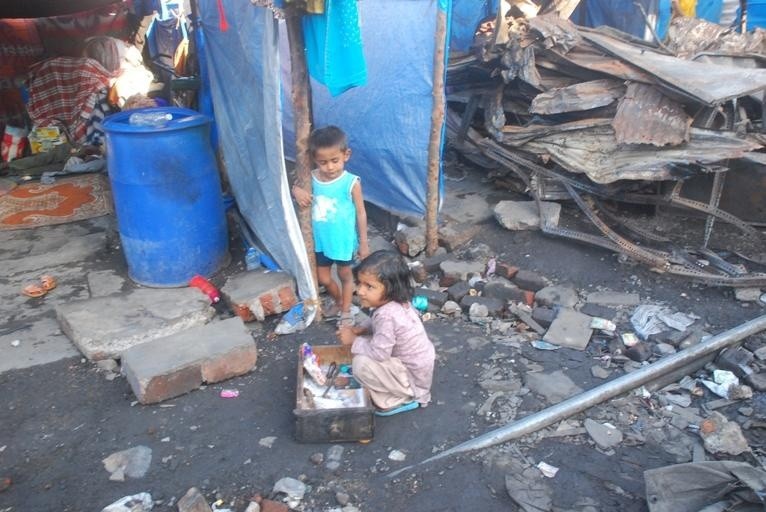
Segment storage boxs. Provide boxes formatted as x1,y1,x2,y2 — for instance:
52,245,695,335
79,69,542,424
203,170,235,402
293,343,375,443
27,126,67,154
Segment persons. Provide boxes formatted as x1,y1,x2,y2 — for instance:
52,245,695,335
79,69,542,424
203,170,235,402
336,248,436,414
289,126,370,327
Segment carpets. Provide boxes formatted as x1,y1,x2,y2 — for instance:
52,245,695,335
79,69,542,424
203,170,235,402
0,176,114,231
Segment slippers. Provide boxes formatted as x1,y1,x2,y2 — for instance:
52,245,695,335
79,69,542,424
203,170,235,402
373,401,419,417
22,275,55,297
323,302,355,328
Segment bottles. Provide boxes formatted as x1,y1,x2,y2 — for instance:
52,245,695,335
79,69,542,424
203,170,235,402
187,274,221,304
243,247,261,272
127,110,173,129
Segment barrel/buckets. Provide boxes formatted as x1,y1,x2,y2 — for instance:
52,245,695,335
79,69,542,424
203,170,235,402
100,105,231,289
